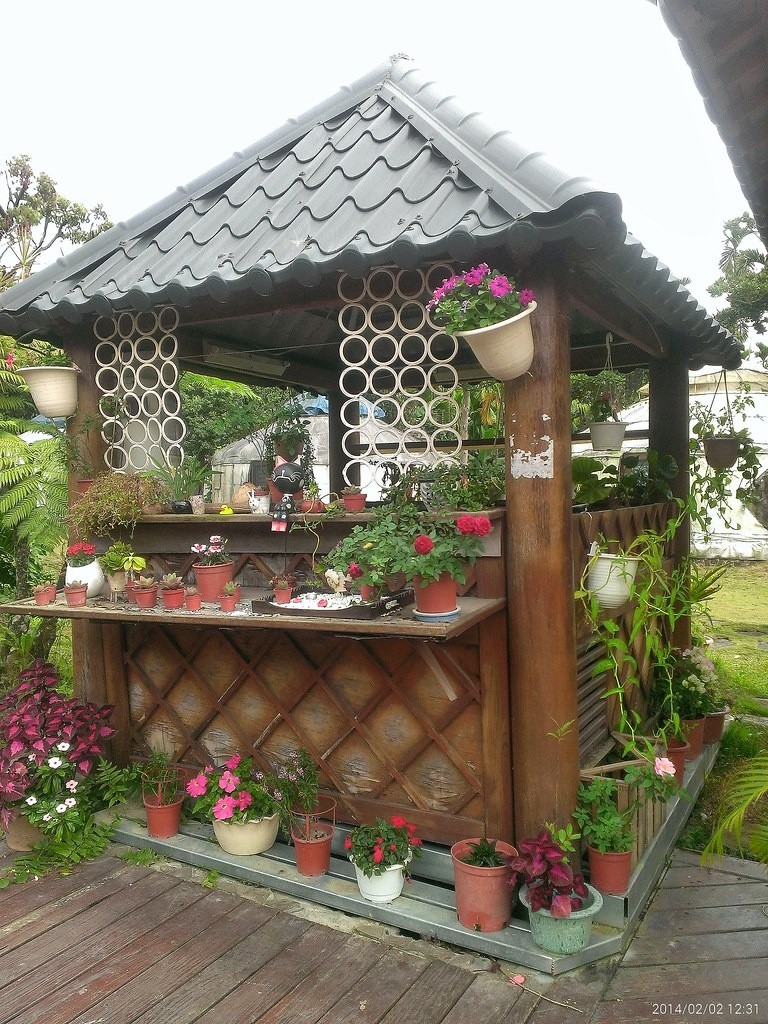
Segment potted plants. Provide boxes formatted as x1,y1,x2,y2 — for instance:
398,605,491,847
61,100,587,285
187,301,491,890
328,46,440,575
32,395,413,609
225,582,240,603
691,384,763,536
142,449,224,514
134,745,189,840
449,776,645,954
218,582,236,612
50,414,103,498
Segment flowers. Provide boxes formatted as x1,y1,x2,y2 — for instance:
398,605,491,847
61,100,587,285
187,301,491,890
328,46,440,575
5,344,78,368
591,393,621,421
0,656,425,884
312,504,495,594
572,477,729,801
422,259,536,335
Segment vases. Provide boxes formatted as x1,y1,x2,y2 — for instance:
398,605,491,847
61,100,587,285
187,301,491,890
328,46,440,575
6,803,49,850
65,557,104,597
288,795,339,877
14,366,230,568
588,551,639,609
413,570,457,612
349,846,414,903
192,560,236,602
455,289,537,380
267,477,303,502
358,579,379,600
587,421,629,453
202,807,278,853
645,702,731,792
